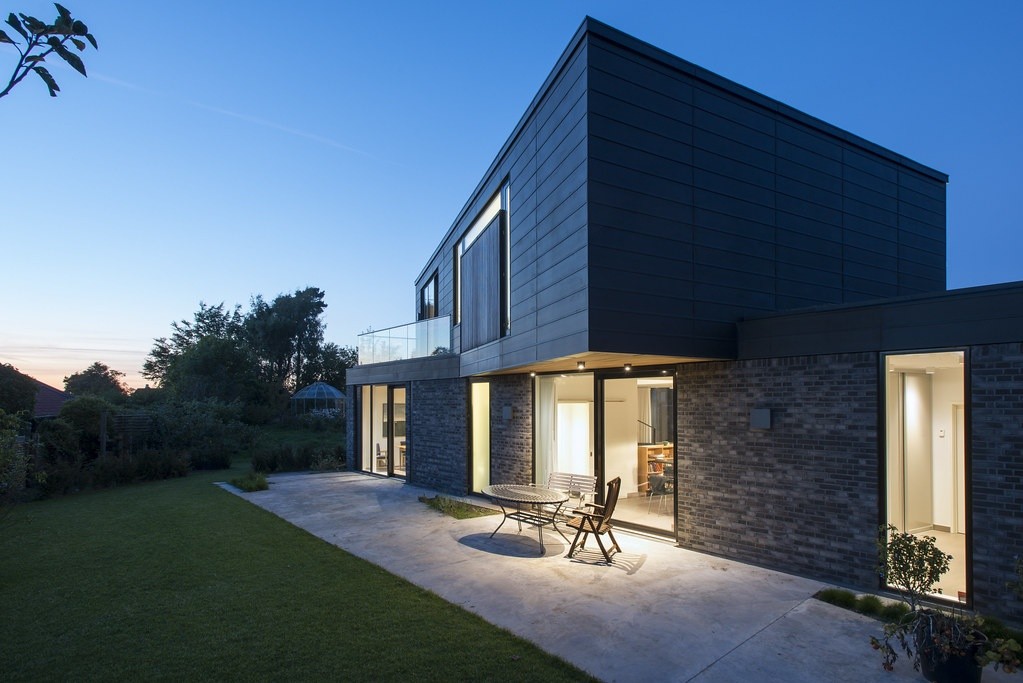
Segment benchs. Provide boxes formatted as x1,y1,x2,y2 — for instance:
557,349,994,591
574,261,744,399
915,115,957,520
529,472,598,530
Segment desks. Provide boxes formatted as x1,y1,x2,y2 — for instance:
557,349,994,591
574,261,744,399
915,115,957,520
483,484,570,554
399,445,406,469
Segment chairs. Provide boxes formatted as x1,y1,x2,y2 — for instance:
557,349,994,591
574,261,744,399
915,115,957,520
647,466,674,514
376,444,387,469
401,441,406,465
566,476,622,565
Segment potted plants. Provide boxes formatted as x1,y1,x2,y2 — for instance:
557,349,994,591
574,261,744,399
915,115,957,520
869,608,1021,683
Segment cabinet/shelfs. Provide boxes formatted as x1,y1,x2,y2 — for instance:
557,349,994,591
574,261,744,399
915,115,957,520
637,445,674,493
